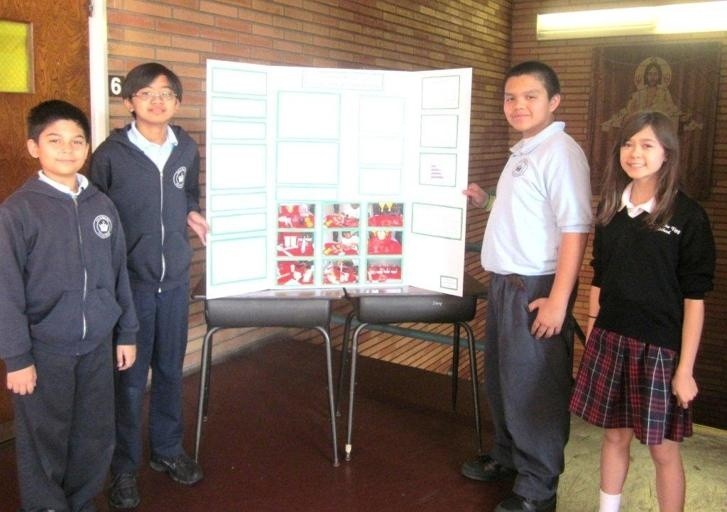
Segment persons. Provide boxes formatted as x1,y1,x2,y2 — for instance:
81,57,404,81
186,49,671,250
341,231,358,250
566,110,718,512
602,55,695,125
460,56,594,512
87,61,210,510
0,98,138,512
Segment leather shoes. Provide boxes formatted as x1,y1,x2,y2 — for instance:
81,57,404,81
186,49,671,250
494,493,557,512
456,454,516,483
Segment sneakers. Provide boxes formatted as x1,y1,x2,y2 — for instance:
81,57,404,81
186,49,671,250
107,470,141,510
149,445,203,485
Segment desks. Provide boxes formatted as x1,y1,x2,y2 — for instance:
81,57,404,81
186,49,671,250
335,270,489,462
191,274,340,468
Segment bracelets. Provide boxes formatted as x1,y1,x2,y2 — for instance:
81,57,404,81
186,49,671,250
587,315,598,318
485,194,496,213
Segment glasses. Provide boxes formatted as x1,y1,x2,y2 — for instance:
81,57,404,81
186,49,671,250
131,89,178,100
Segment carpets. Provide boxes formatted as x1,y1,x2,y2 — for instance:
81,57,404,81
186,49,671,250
554,412,727,512
